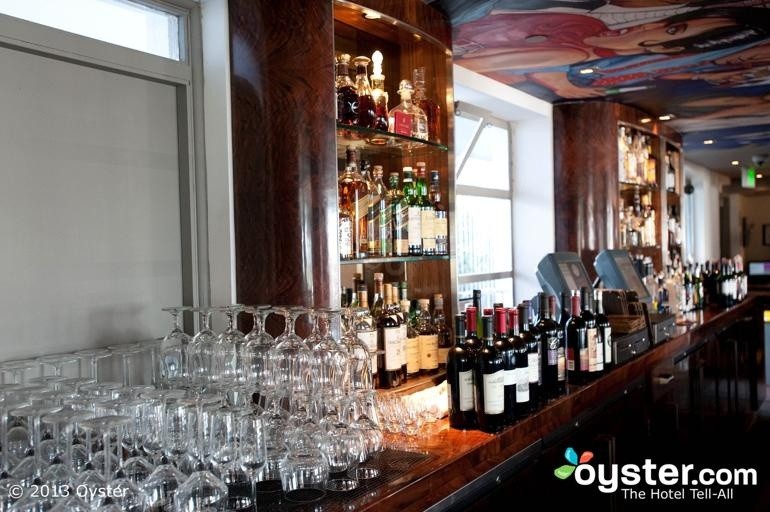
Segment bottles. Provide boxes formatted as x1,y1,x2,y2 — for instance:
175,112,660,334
446,287,613,433
338,147,449,257
340,265,448,380
613,121,752,329
334,48,446,147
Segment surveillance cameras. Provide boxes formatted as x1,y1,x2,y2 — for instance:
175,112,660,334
751,155,769,168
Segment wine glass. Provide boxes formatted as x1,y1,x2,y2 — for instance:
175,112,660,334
0,300,390,512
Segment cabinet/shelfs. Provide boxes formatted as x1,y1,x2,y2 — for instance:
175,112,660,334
229,2,456,402
549,95,686,286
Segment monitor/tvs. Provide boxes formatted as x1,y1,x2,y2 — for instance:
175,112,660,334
536,252,594,310
594,248,652,302
